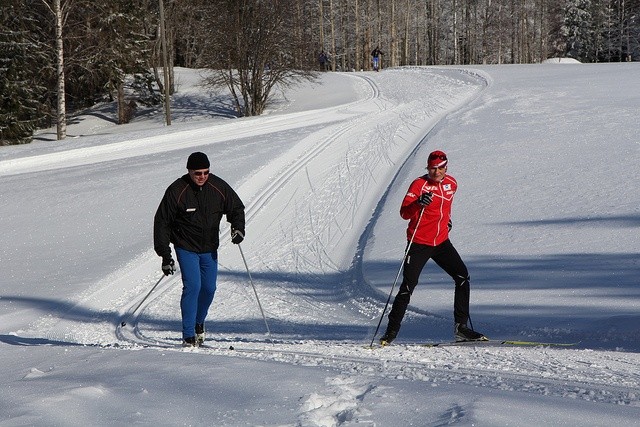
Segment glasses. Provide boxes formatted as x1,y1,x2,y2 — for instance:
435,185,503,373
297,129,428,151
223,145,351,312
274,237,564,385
194,171,209,175
429,155,447,160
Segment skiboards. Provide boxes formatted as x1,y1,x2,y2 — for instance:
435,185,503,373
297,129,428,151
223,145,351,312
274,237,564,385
361,338,582,348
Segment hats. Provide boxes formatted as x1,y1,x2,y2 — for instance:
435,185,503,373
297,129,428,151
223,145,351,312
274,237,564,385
427,151,448,168
186,152,210,169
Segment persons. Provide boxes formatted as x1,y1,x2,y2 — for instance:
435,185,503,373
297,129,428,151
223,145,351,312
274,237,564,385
153,152,245,349
370,46,384,72
318,50,330,72
379,150,485,347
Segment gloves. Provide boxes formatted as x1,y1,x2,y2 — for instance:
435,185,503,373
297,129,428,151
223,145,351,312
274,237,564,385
418,191,433,207
447,219,452,232
162,255,176,276
231,222,245,244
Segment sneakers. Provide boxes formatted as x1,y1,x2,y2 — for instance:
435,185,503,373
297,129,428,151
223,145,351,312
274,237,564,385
195,323,205,342
182,336,199,348
455,327,489,342
380,334,397,346
